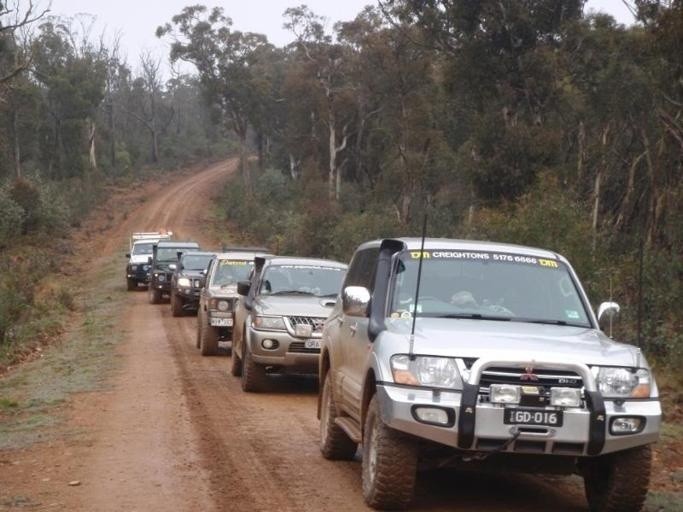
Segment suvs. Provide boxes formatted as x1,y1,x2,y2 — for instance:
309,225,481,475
169,250,213,317
230,253,350,394
315,239,661,510
146,243,202,304
196,253,283,356
125,231,174,291
